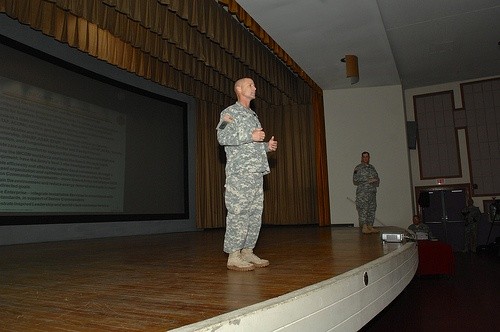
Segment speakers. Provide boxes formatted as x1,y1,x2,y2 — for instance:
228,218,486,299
422,188,467,248
407,121,417,149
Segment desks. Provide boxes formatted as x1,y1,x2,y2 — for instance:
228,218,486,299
417,240,454,274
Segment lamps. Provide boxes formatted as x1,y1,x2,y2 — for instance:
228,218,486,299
340,55,360,78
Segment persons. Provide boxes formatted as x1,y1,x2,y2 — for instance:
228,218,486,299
408,215,433,239
458,199,482,253
216,78,278,273
353,152,382,234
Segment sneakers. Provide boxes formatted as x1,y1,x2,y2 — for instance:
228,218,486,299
240,248,269,267
368,224,380,232
362,224,370,233
227,251,255,271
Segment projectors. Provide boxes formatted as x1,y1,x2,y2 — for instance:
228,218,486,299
381,228,409,242
